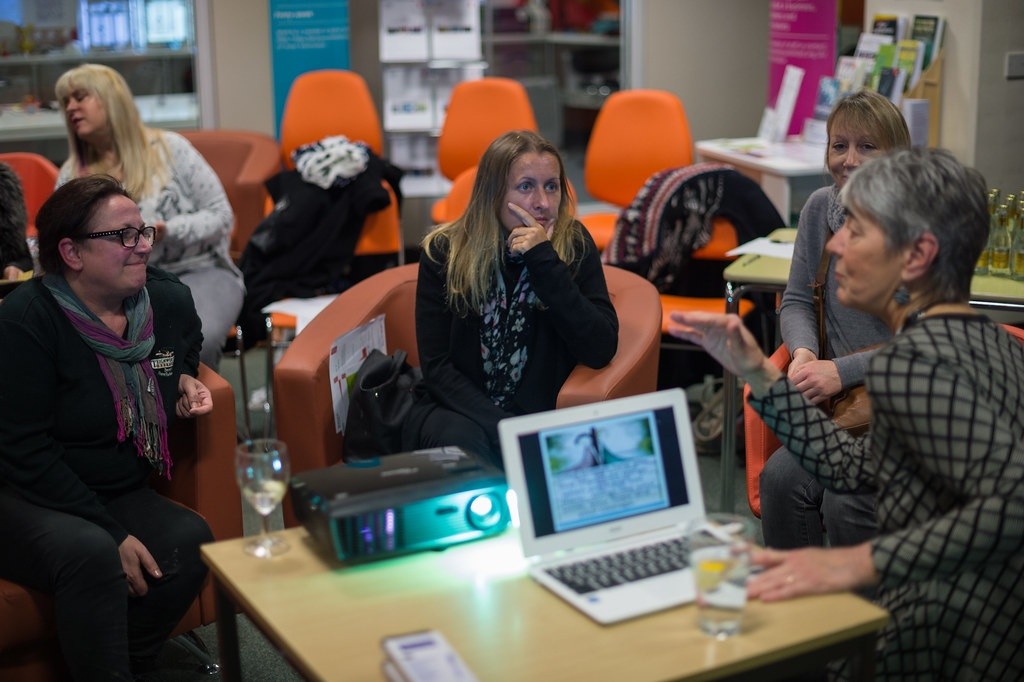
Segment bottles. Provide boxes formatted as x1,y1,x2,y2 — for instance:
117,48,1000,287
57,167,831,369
973,188,999,275
1012,191,1024,279
1005,195,1017,243
991,204,1011,274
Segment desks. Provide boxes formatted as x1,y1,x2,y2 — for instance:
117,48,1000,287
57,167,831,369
201,478,891,682
694,137,830,226
720,227,1024,515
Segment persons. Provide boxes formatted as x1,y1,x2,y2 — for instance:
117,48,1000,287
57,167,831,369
0,171,214,682
53,63,248,377
759,88,912,550
666,146,1024,682
401,130,620,471
0,163,36,303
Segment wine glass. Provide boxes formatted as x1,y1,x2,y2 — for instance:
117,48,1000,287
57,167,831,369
234,440,291,557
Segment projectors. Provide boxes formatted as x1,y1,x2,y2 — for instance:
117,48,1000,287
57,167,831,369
290,445,512,566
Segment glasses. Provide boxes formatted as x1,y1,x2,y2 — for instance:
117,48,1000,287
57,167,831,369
82,226,156,248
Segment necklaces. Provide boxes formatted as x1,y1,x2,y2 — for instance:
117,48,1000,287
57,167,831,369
894,295,965,335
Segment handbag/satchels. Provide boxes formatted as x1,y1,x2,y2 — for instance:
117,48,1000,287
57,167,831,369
344,349,420,464
683,376,725,455
826,341,885,430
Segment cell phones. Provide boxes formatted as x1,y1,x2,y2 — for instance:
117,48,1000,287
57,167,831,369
381,630,477,682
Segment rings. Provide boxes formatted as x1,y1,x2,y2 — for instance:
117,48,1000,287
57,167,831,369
785,575,795,584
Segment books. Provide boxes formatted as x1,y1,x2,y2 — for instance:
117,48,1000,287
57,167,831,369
814,13,948,123
377,0,484,198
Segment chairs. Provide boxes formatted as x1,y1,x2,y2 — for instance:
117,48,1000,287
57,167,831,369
0,68,1024,682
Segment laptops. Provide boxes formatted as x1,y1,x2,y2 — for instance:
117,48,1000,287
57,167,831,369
497,388,737,625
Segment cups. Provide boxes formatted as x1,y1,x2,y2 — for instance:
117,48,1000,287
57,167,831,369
688,514,754,635
